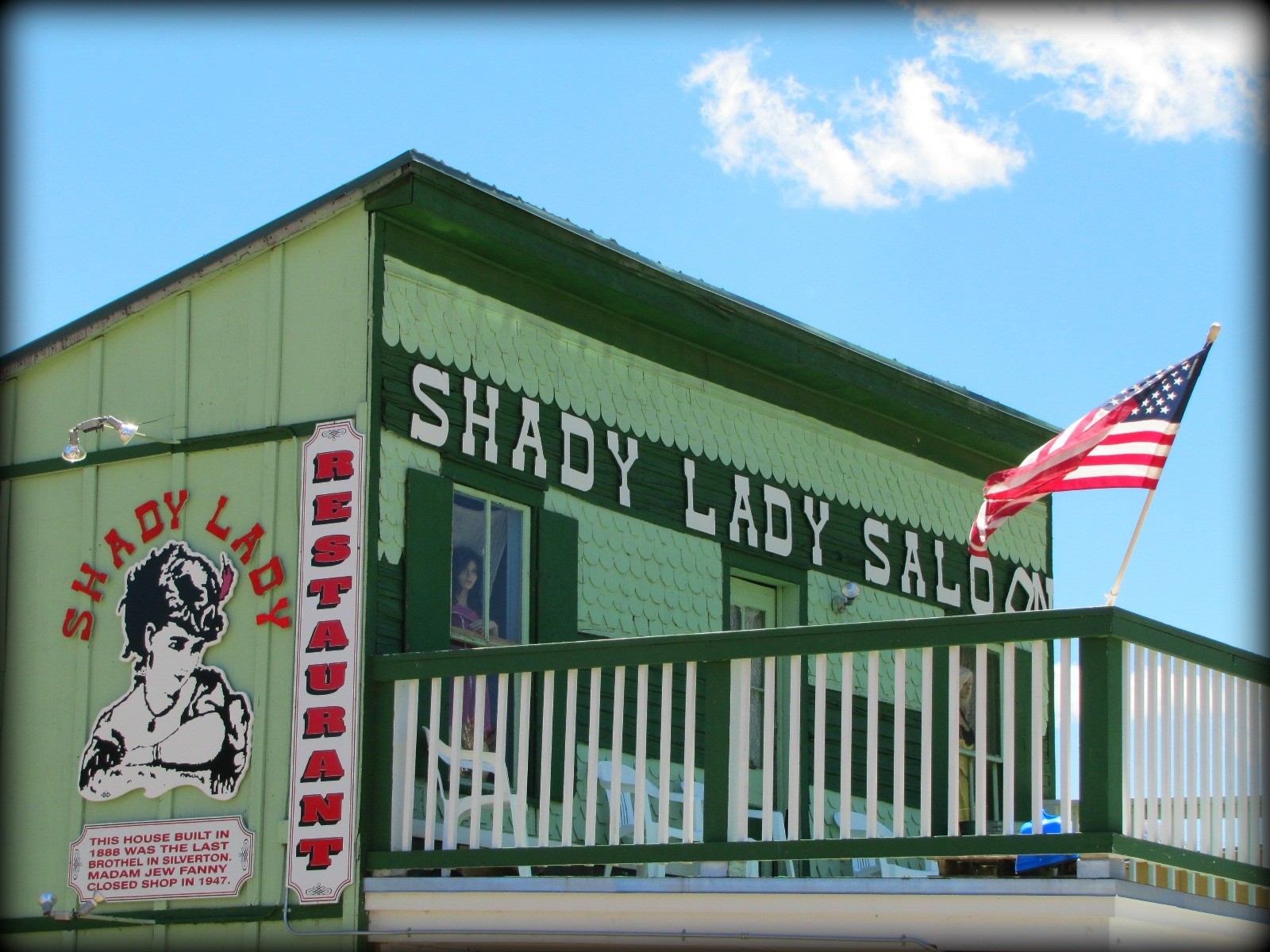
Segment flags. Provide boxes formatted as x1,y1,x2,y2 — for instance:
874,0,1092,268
967,343,1213,559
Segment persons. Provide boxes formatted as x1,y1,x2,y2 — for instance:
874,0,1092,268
959,664,977,823
450,546,498,750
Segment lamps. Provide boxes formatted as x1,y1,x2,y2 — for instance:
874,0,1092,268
38,890,156,924
832,582,860,613
60,415,180,462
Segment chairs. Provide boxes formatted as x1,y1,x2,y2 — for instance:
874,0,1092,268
422,725,532,877
680,781,795,878
833,811,939,877
598,760,702,878
